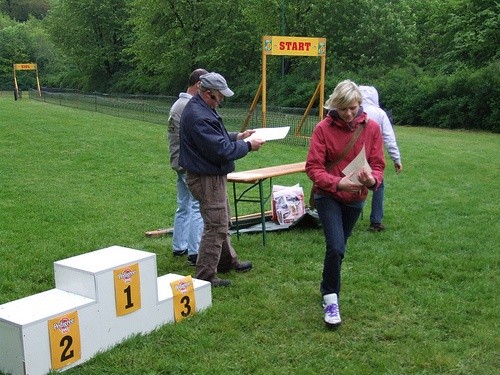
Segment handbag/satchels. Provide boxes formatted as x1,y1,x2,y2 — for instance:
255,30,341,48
309,187,317,209
270,183,306,226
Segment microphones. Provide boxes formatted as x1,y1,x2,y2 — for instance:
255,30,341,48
211,95,222,108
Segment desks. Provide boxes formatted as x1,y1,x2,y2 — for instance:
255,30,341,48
227,162,306,247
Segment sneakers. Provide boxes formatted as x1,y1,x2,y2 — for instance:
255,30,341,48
208,276,230,287
227,259,252,272
322,293,342,325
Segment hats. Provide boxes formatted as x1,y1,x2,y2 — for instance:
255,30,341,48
199,72,234,97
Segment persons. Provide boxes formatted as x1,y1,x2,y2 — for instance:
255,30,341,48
167,68,212,266
305,79,386,327
178,73,266,288
356,84,404,232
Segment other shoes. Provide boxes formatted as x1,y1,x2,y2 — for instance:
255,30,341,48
369,222,386,231
172,248,187,256
187,254,198,265
